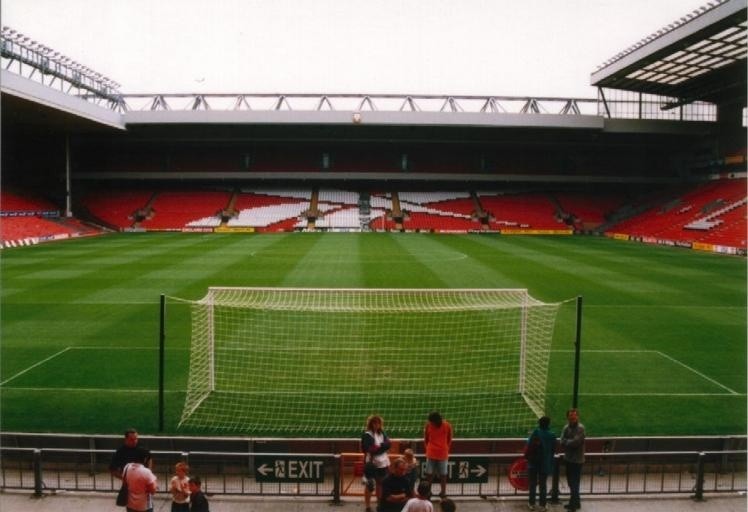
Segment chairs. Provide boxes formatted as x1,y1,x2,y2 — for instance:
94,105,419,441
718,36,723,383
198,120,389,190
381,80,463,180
2,175,747,256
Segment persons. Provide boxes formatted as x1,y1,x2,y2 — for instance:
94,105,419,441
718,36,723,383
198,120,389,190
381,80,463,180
377,457,416,512
423,412,453,503
170,462,190,512
400,480,433,512
122,446,158,512
360,416,391,512
560,407,585,512
188,477,209,512
112,430,144,481
404,449,419,489
440,499,457,512
525,416,557,511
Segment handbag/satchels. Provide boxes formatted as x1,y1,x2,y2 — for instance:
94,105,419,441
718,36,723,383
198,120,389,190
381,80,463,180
116,482,130,508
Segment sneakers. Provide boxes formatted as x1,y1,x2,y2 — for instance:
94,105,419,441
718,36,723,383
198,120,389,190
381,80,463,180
527,502,549,511
564,504,581,512
